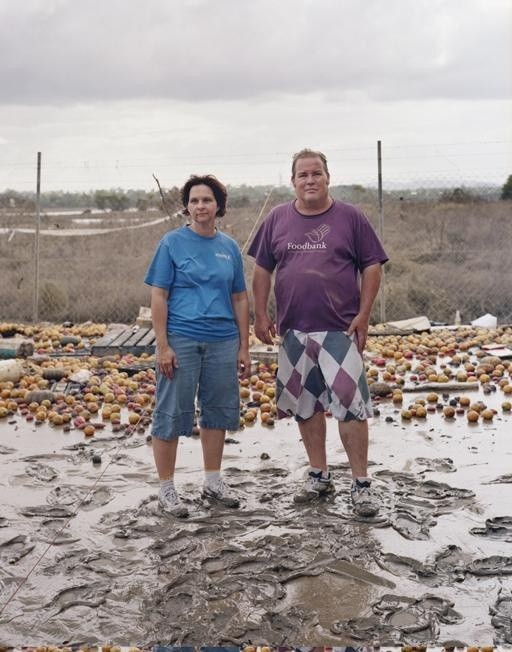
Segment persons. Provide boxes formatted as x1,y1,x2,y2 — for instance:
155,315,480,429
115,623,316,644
246,146,389,516
144,174,251,517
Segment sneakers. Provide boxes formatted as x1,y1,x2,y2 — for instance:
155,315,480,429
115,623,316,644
204,482,240,507
160,490,188,517
351,476,380,515
294,469,332,503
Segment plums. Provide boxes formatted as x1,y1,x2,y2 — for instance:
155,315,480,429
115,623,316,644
0,319,512,436
